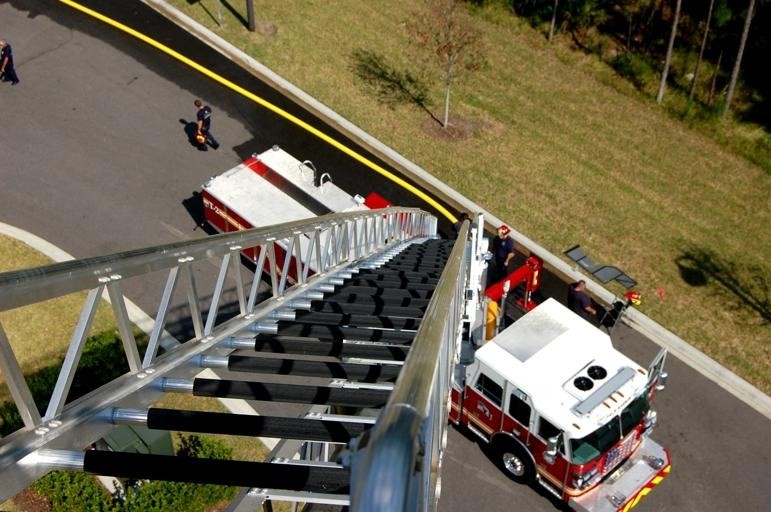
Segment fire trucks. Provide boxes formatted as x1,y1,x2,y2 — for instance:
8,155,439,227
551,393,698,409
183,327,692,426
0,145,674,512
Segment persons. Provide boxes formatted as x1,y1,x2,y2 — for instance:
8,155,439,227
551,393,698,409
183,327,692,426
491,225,515,278
0,40,19,85
602,301,624,327
568,280,597,318
194,100,219,151
453,213,471,240
